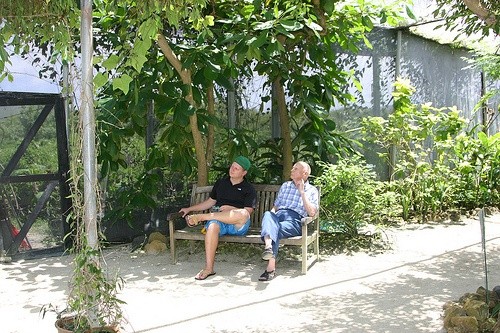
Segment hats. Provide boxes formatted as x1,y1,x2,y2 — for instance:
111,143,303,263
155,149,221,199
235,154,251,171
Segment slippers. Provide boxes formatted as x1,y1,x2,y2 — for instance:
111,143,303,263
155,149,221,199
185,213,201,228
194,270,216,280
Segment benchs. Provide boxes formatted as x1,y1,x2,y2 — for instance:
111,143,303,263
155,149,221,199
167,183,322,274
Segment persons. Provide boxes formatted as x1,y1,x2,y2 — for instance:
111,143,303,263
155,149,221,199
258,161,320,282
179,156,256,280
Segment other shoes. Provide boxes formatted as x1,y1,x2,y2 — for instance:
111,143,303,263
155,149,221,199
259,269,275,280
262,248,276,261
